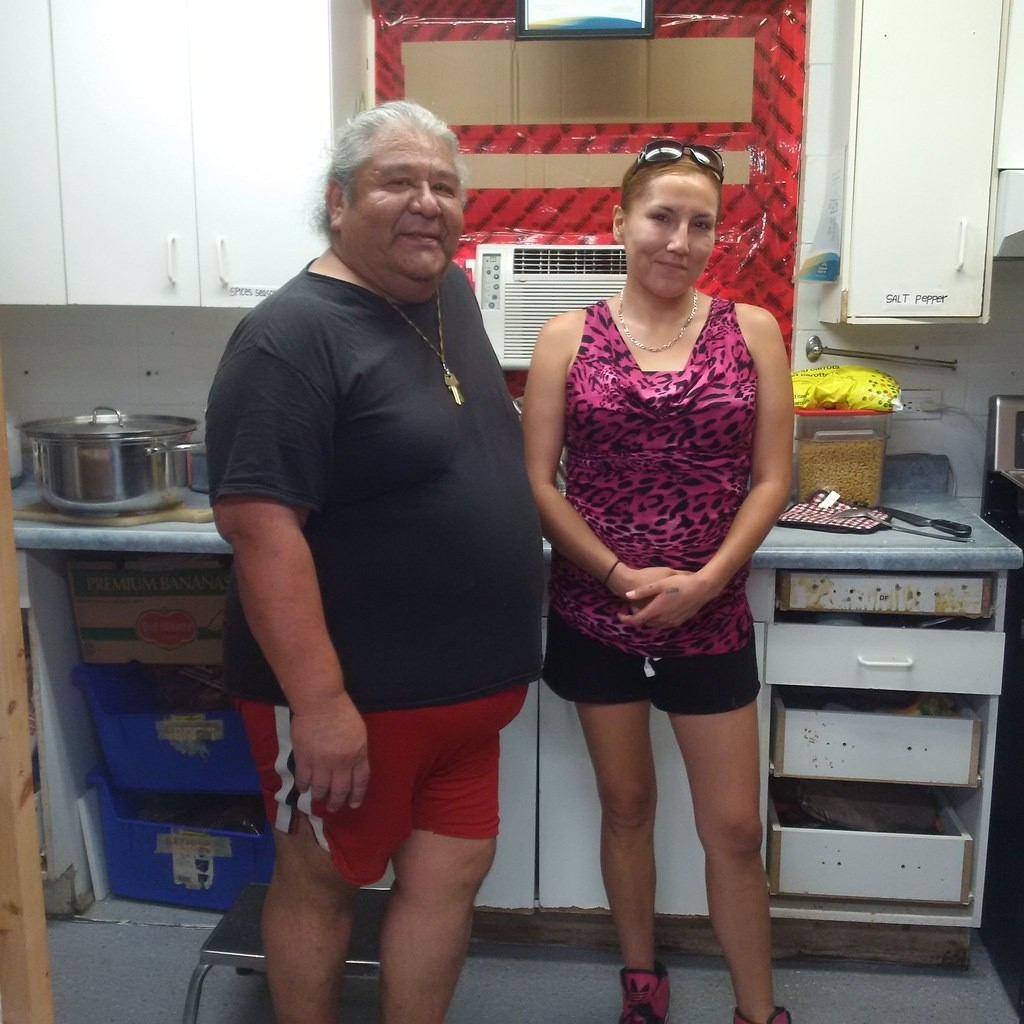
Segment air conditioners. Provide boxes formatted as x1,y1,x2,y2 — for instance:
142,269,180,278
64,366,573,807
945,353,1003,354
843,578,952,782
476,243,628,371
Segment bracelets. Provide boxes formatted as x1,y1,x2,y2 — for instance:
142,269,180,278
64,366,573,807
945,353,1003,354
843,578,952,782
604,561,621,586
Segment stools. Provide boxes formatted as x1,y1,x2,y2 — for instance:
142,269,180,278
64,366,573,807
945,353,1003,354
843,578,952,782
181,880,396,1024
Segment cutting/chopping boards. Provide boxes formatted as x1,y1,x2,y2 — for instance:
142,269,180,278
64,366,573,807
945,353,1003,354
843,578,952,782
13,499,217,526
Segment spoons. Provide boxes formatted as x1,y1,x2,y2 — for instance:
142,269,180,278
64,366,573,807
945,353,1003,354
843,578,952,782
837,508,968,542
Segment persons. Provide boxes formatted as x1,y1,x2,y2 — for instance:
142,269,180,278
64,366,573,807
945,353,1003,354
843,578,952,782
205,101,542,1024
522,142,794,1024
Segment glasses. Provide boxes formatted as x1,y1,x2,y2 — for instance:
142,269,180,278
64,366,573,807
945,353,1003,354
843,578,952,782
623,139,725,188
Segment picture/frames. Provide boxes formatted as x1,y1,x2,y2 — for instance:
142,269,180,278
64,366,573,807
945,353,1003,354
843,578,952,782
514,0,655,40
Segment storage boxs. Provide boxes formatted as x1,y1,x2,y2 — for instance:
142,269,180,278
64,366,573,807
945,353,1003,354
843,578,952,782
62,552,274,914
793,408,893,509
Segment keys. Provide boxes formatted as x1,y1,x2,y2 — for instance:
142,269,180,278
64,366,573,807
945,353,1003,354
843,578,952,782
444,372,464,405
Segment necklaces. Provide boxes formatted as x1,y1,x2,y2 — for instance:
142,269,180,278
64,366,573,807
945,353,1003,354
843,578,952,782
618,288,698,352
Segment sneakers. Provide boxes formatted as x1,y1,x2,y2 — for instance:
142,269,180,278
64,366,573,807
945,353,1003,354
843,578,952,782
733,1008,791,1024
618,962,670,1024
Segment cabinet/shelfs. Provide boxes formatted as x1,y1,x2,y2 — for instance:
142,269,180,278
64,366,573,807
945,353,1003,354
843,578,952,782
763,569,1009,927
358,621,763,916
1,0,368,309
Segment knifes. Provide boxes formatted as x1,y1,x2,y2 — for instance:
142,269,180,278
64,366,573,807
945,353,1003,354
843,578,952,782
875,506,972,537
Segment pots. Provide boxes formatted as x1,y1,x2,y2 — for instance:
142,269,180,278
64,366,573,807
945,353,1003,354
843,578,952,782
14,406,205,518
